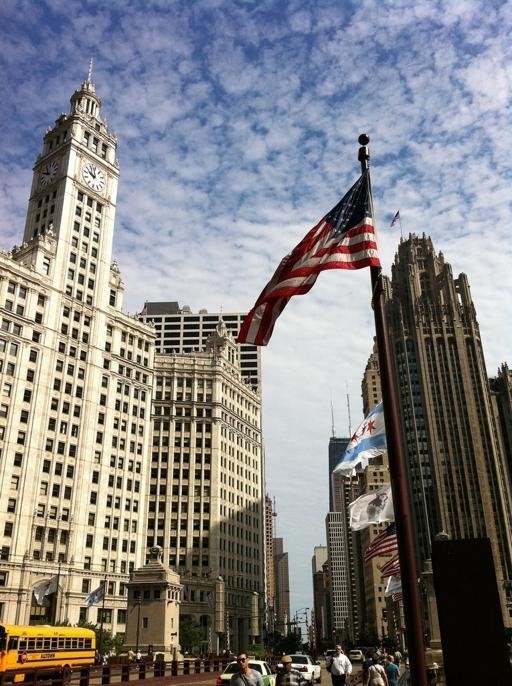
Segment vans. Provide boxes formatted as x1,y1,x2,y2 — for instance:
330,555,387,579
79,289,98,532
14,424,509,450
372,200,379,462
349,647,375,664
324,649,344,670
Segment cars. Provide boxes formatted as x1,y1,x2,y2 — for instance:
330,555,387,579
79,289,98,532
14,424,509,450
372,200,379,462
216,660,278,686
276,654,322,686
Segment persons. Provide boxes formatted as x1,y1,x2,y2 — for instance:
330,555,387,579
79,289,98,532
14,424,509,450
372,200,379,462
219,648,231,655
94,649,111,666
330,645,352,686
361,646,410,686
136,649,142,662
230,652,265,686
182,649,191,655
128,647,135,664
275,655,308,686
147,642,153,655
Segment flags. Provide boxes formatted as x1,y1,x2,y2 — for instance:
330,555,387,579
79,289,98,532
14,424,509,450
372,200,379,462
333,401,388,477
347,482,395,531
33,574,58,605
362,523,398,562
84,580,108,608
385,572,402,597
378,553,401,577
238,171,383,346
390,211,399,227
391,592,403,602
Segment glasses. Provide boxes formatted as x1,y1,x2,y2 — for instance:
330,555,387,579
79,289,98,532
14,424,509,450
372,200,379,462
237,657,248,664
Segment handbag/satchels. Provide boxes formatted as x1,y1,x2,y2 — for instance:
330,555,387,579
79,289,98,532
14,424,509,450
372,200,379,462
326,655,334,672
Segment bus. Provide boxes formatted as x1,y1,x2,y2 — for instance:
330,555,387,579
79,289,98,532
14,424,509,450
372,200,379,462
0,624,97,682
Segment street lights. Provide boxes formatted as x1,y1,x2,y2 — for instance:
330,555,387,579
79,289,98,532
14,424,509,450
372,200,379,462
137,594,142,649
292,608,310,631
273,590,290,640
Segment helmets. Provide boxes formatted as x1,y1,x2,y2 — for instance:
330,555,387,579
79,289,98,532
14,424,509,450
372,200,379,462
280,655,292,663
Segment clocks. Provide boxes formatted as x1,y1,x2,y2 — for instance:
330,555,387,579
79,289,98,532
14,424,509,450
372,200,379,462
37,157,60,188
82,162,106,192
